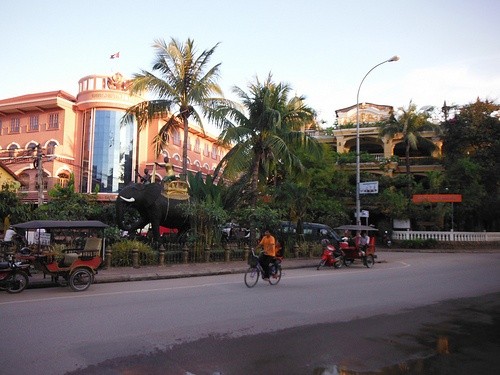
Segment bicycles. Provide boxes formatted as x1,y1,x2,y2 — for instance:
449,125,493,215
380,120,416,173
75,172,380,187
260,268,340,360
244,247,283,289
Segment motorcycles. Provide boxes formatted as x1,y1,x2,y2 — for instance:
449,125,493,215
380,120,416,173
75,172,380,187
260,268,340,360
0,253,33,294
316,228,347,271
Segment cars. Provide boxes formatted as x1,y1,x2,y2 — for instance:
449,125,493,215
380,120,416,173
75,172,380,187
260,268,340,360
218,223,251,242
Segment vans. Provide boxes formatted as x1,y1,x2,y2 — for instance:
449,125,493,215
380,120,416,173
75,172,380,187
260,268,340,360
122,219,180,242
275,221,342,247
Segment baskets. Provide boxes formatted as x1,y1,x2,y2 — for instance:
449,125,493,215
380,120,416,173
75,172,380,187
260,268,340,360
247,255,258,266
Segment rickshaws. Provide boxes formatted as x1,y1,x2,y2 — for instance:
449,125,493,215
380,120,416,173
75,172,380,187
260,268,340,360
333,224,379,269
3,219,109,294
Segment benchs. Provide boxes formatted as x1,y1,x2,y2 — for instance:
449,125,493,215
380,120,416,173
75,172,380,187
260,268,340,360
61,237,105,265
353,237,375,254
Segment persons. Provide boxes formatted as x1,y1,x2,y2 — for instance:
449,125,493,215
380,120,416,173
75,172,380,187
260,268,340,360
155,156,176,184
254,228,276,279
273,238,284,278
135,168,151,183
358,232,370,256
4,225,26,255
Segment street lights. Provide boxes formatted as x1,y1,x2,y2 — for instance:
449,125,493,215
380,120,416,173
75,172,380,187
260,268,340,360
356,55,400,224
